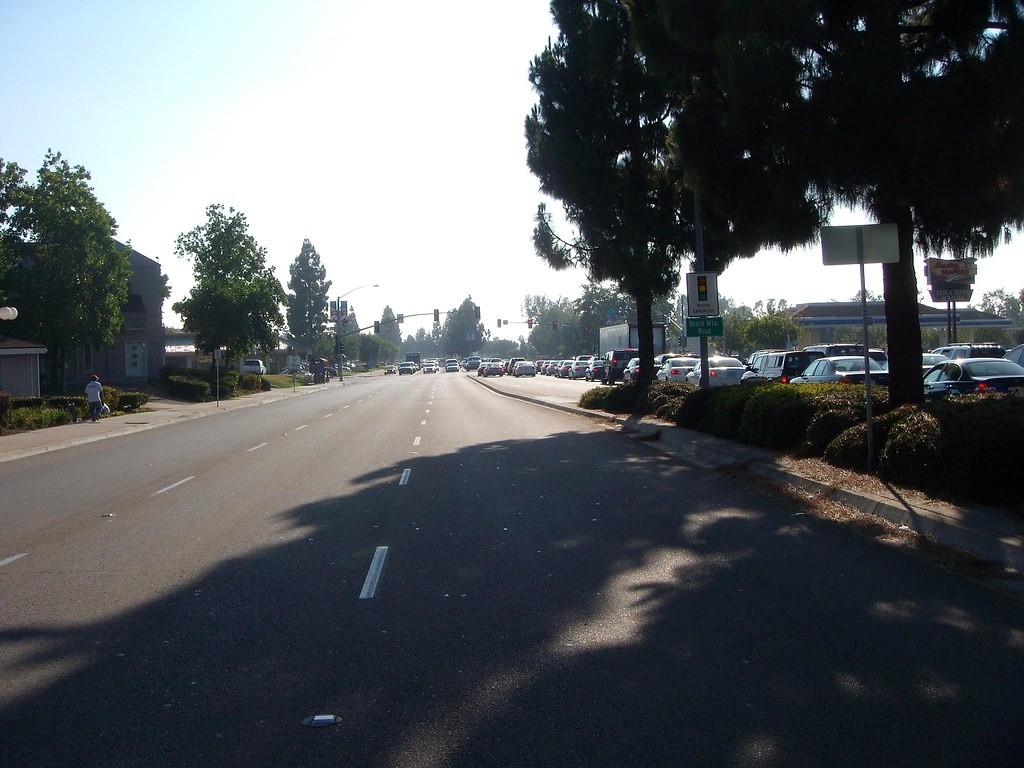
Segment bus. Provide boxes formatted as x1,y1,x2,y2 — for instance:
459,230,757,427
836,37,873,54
406,352,423,370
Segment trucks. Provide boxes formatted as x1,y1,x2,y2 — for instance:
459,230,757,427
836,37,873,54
593,323,666,362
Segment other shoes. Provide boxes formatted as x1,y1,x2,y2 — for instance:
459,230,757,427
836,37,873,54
92,416,99,421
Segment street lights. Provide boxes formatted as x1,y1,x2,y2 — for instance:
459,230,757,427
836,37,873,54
337,284,379,381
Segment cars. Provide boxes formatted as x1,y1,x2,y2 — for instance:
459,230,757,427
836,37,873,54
384,365,396,375
461,355,537,378
445,358,460,372
540,342,1023,394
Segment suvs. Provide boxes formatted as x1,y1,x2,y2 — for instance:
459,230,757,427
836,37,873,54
423,359,440,374
244,359,267,375
398,362,419,375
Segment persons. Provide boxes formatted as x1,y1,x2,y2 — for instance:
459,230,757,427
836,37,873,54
84,374,105,423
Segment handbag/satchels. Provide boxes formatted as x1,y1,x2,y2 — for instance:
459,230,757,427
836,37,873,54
101,403,110,414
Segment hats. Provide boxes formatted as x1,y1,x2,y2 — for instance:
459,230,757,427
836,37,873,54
91,375,99,380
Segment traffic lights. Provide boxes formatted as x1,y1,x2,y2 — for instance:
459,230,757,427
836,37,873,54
528,320,532,328
583,326,589,333
553,320,557,330
498,319,501,327
